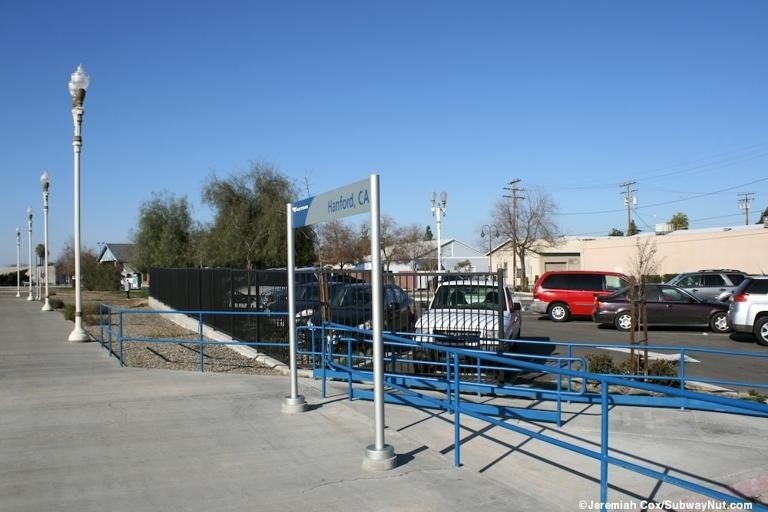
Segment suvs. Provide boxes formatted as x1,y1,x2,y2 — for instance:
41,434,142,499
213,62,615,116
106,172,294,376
726,273,768,346
661,271,746,305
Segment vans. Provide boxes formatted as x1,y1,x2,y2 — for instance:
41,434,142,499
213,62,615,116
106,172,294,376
531,271,632,322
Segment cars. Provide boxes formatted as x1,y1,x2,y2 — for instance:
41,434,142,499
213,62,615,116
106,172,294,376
592,283,733,334
414,280,522,350
229,266,419,339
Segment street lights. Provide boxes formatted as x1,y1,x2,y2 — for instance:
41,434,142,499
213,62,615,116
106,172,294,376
481,224,499,272
25,206,35,300
67,64,89,342
430,191,447,288
40,170,54,311
15,225,22,297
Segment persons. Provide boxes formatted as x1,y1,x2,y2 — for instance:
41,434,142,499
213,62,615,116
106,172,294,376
124,279,130,299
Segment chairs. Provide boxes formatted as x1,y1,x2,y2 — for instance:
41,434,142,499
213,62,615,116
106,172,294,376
484,291,498,304
451,291,468,307
